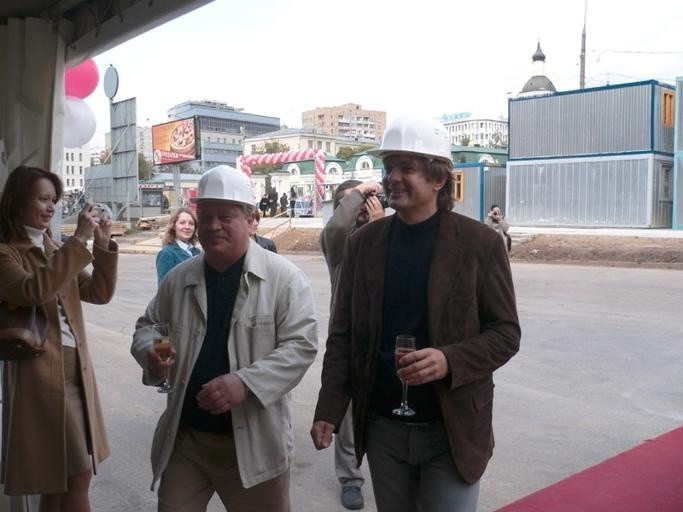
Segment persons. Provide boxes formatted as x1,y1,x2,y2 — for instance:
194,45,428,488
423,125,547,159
155,208,201,283
321,179,386,511
309,115,522,512
245,207,277,253
268,186,278,217
288,187,296,218
260,194,268,216
487,205,509,246
0,166,119,512
280,193,288,217
131,164,320,512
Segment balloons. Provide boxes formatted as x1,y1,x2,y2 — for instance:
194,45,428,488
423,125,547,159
65,58,99,99
64,96,96,149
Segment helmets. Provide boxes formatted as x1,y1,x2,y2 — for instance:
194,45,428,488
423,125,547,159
190,165,256,208
366,116,453,169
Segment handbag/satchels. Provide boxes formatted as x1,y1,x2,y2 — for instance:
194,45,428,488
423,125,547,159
0,297,47,360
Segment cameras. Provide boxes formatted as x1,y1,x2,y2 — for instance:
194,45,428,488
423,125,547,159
90,206,105,222
493,212,497,216
364,192,389,209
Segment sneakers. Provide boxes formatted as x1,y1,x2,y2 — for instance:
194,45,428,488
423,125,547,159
341,485,363,510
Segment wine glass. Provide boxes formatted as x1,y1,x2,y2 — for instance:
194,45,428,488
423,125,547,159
390,334,417,417
151,321,176,394
